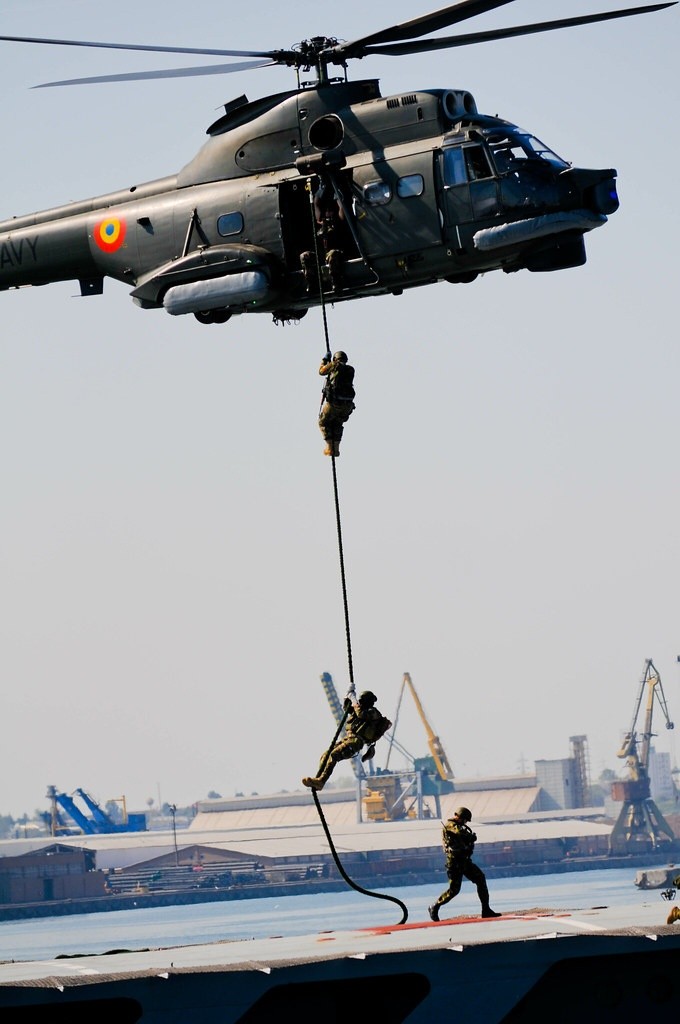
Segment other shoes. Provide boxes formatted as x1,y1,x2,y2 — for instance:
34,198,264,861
308,777,324,790
303,778,312,787
305,286,316,293
482,910,502,918
331,282,343,292
429,904,440,922
335,450,340,457
324,448,334,455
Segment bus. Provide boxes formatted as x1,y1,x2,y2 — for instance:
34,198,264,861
40,785,149,839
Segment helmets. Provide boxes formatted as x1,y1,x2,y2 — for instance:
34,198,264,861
359,691,378,702
333,351,348,362
455,808,473,822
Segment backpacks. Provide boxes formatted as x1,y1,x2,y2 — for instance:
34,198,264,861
349,706,392,763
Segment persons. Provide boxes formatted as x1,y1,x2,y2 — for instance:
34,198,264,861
301,690,383,789
318,351,357,458
667,906,680,924
428,807,502,923
494,150,515,174
299,188,347,293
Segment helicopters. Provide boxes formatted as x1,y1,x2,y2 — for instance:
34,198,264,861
2,0,676,328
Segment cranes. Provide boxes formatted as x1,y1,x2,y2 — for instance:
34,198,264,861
570,733,593,809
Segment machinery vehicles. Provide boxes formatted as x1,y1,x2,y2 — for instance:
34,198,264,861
322,662,457,823
604,655,674,853
